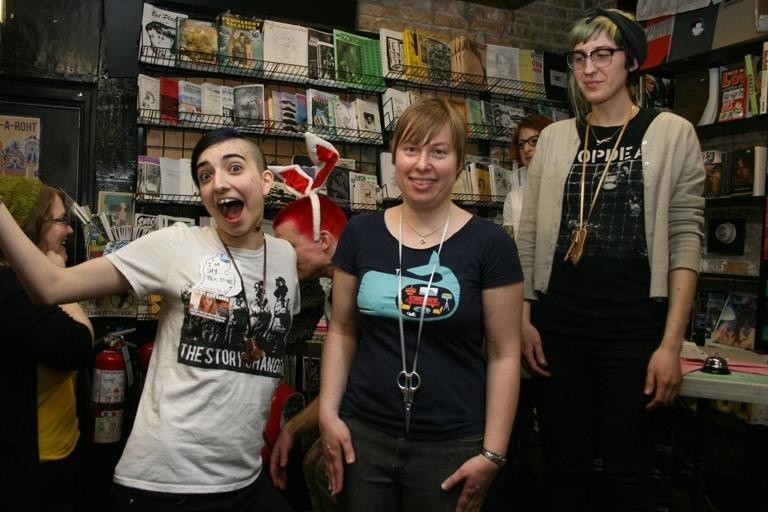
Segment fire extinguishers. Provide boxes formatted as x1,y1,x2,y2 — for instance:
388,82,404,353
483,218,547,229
139,342,154,397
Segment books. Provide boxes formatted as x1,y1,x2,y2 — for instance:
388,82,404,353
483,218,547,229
136,1,570,210
631,41,767,353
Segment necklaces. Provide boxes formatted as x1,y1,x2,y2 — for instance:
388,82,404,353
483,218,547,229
589,123,623,145
403,216,447,244
219,236,269,360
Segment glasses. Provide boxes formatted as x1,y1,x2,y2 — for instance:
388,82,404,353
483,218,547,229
514,134,543,150
40,216,69,225
564,46,628,72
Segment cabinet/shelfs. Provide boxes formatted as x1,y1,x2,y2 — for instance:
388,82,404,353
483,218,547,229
638,33,768,356
133,7,575,334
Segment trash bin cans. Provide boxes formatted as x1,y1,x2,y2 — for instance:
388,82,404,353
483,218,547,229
93,327,137,445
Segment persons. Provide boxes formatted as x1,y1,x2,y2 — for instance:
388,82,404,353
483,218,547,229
503,115,555,242
1,125,302,512
268,131,347,491
0,175,94,512
318,97,524,512
505,7,708,512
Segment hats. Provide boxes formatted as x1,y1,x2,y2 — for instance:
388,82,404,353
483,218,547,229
0,175,61,242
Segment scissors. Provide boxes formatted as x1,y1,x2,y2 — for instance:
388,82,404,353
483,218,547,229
396,371,421,432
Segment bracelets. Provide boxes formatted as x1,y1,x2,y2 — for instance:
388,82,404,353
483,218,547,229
481,449,507,467
